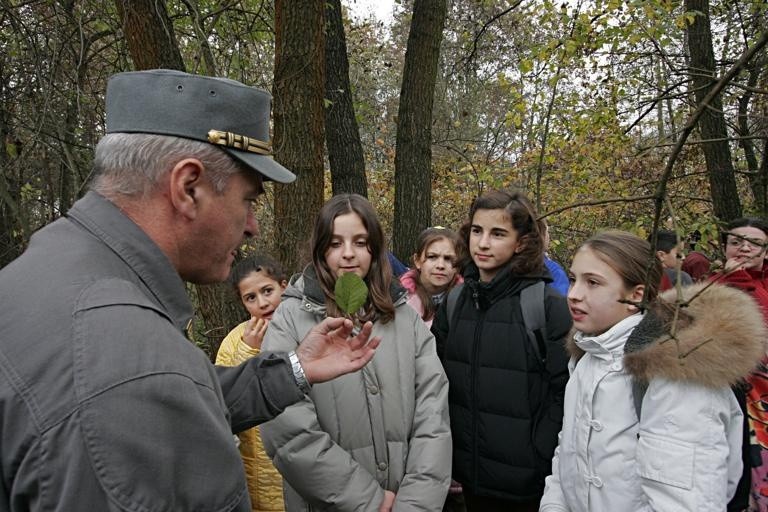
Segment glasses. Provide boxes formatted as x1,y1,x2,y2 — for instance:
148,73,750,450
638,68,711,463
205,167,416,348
727,237,763,248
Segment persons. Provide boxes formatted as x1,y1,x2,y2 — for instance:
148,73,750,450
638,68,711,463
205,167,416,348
1,67,383,512
218,187,766,512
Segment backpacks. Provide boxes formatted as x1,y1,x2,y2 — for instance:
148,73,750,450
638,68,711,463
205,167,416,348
632,368,752,511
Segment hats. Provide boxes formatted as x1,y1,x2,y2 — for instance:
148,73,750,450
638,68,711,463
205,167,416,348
105,69,297,184
